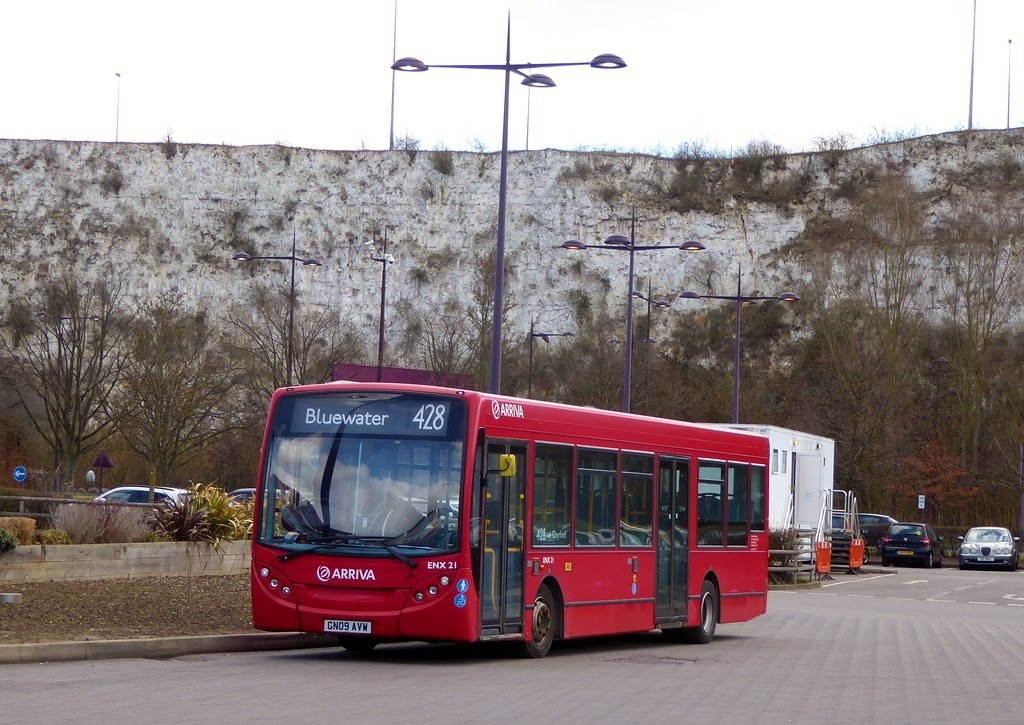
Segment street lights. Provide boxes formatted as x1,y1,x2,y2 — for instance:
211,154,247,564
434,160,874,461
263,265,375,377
678,262,800,422
527,315,572,399
233,228,323,386
370,226,394,382
560,235,705,412
608,275,671,414
392,8,627,403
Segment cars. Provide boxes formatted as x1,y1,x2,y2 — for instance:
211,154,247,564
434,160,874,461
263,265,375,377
882,522,944,569
227,488,290,504
852,513,899,547
957,527,1021,572
94,486,193,509
832,516,870,565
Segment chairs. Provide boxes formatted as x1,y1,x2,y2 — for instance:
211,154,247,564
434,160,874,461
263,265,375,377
559,488,741,526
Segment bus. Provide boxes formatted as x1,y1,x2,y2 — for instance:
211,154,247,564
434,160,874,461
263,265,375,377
250,381,771,659
